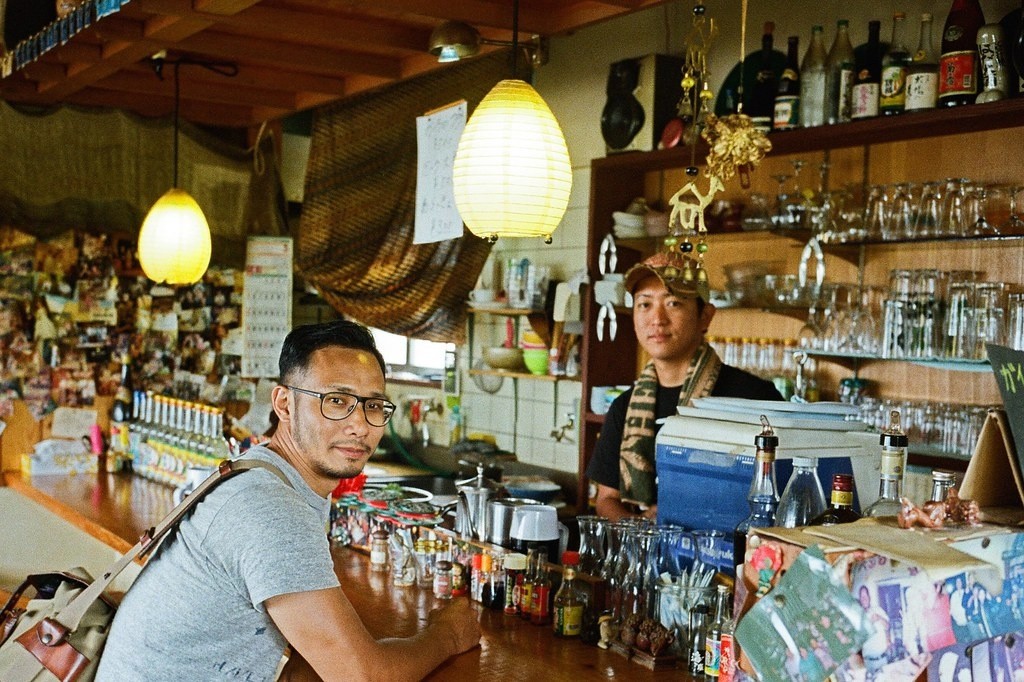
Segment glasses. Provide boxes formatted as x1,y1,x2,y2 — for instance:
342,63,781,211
283,385,398,427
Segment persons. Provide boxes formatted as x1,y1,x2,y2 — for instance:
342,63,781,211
93,319,481,682
587,252,784,520
760,571,1024,682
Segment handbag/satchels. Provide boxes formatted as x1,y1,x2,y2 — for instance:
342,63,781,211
0,568,120,682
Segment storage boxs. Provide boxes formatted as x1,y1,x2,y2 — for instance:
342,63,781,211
650,417,885,568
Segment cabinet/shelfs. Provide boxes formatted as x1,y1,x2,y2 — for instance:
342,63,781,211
577,93,1024,519
465,297,581,382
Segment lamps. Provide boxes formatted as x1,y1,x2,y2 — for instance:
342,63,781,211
428,21,549,70
454,0,571,241
141,63,213,284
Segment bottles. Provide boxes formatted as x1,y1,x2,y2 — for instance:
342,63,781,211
0,0,1023,682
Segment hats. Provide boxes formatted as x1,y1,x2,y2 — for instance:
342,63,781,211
622,251,710,302
